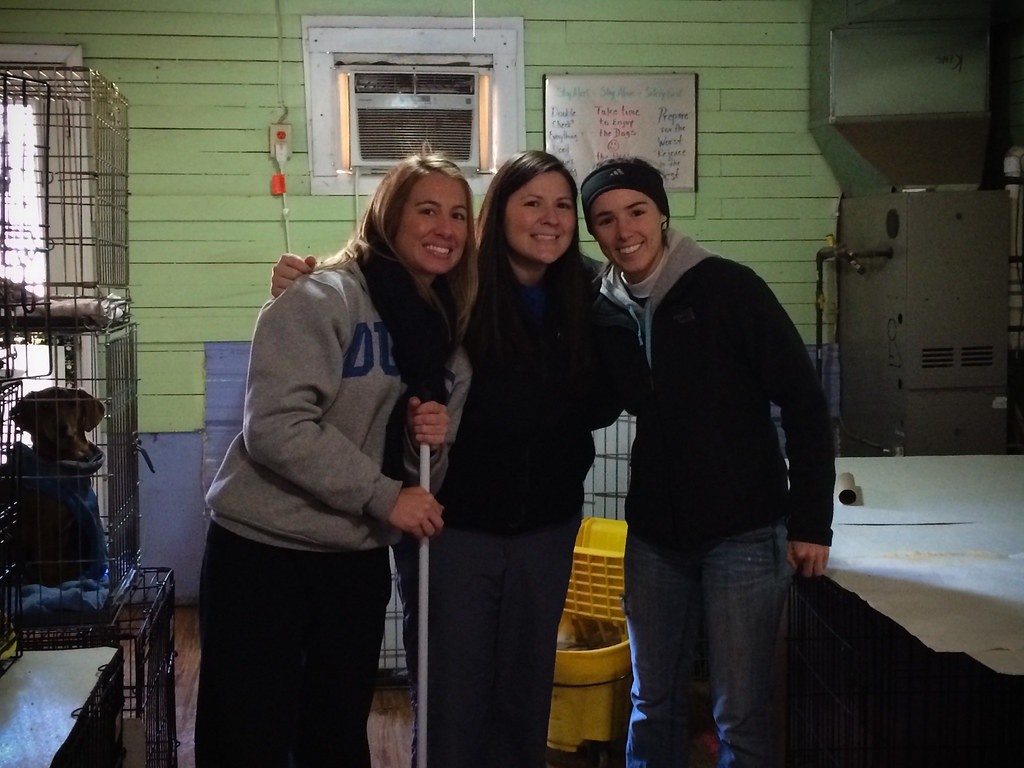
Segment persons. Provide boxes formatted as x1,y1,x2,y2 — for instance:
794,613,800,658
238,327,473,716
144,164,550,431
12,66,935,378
579,159,837,767
194,157,480,767
271,150,639,767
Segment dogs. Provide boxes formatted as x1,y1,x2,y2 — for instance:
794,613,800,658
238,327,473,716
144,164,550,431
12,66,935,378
0,387,105,587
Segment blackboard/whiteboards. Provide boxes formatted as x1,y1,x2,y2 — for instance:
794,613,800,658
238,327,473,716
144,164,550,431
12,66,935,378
542,73,699,194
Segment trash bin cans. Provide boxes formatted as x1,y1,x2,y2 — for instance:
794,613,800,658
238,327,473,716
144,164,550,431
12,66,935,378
546,517,632,753
